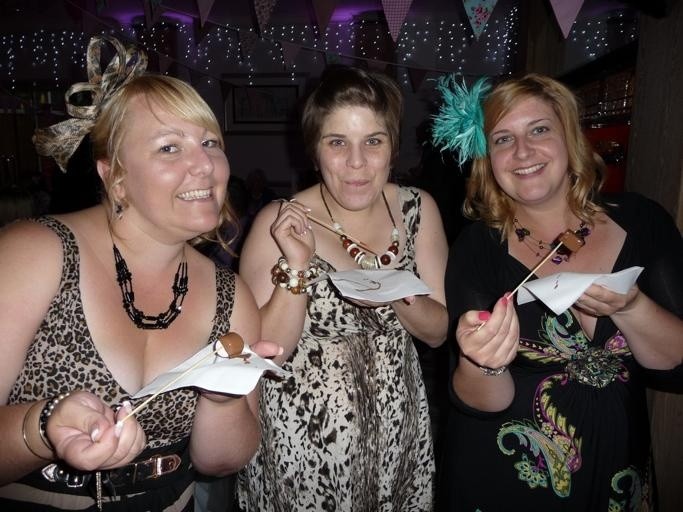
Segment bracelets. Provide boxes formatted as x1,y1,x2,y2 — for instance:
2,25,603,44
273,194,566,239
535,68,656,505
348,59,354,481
462,354,506,377
21,395,56,462
270,255,317,294
38,390,72,450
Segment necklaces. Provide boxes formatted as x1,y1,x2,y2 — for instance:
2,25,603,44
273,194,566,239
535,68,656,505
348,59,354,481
510,209,594,265
318,181,400,270
106,218,189,331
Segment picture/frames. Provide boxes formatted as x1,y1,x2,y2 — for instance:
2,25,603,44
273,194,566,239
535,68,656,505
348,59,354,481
220,73,309,136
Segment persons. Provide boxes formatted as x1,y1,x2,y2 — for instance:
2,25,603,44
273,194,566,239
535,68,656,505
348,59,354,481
232,64,449,511
422,71,683,511
0,34,286,512
